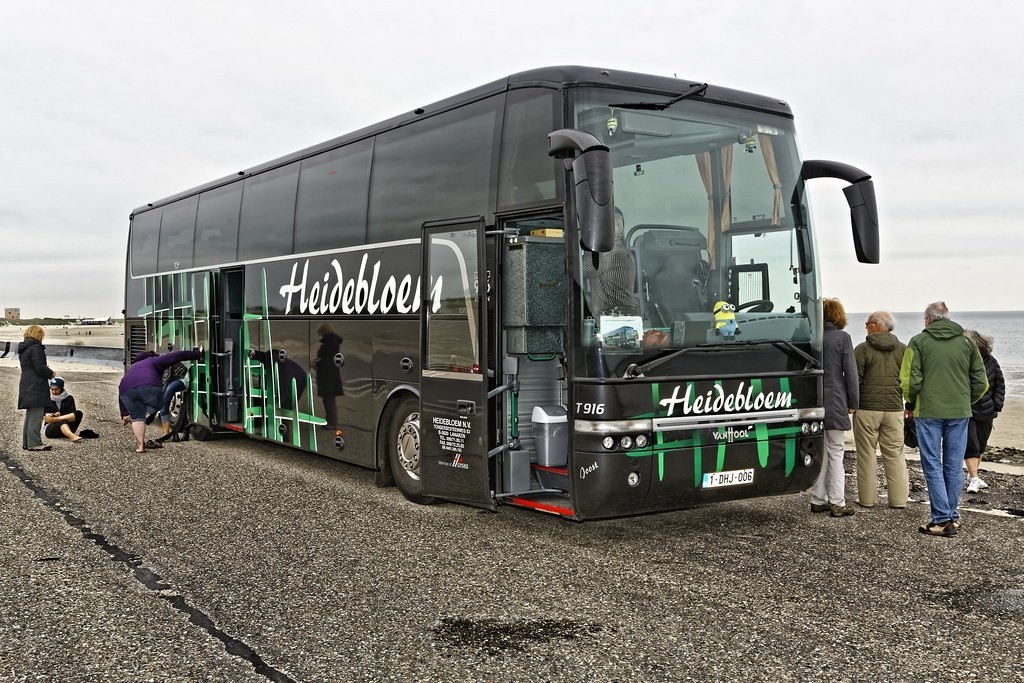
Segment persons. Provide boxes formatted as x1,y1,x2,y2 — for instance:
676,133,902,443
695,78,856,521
962,327,1005,494
118,344,204,453
579,208,641,377
18,325,56,452
43,376,84,442
810,296,856,517
854,310,909,508
311,322,345,430
248,348,307,442
899,301,989,535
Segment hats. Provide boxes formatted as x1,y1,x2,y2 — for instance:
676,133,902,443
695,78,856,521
49,378,64,387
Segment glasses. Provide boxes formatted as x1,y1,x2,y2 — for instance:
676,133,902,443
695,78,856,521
866,321,876,326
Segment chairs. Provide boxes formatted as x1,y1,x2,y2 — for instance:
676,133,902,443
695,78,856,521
624,224,709,328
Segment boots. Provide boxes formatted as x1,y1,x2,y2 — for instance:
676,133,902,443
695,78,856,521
158,423,194,443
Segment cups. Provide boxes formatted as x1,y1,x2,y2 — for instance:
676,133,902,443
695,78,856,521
584,315,595,347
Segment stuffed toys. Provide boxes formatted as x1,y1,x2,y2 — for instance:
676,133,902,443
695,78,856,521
714,301,741,340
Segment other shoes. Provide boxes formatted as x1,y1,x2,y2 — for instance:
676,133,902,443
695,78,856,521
322,424,335,430
24,445,52,450
145,409,160,425
79,429,99,439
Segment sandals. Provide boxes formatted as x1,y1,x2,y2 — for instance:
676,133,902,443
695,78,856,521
145,439,164,448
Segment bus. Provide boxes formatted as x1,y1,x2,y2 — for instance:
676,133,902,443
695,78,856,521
122,62,881,526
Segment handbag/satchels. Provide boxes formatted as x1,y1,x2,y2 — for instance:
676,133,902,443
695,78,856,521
903,416,920,448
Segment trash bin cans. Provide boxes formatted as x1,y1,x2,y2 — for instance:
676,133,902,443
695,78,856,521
530,404,568,468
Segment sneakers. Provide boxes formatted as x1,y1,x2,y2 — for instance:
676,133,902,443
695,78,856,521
920,520,962,535
966,473,988,493
811,502,855,517
